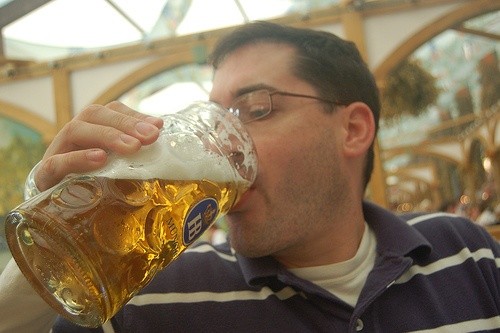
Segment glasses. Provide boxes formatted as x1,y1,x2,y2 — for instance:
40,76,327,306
226,88,349,122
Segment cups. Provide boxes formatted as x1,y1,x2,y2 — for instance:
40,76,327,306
5,101,259,329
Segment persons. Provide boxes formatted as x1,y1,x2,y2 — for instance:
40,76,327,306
0,19,500,333
